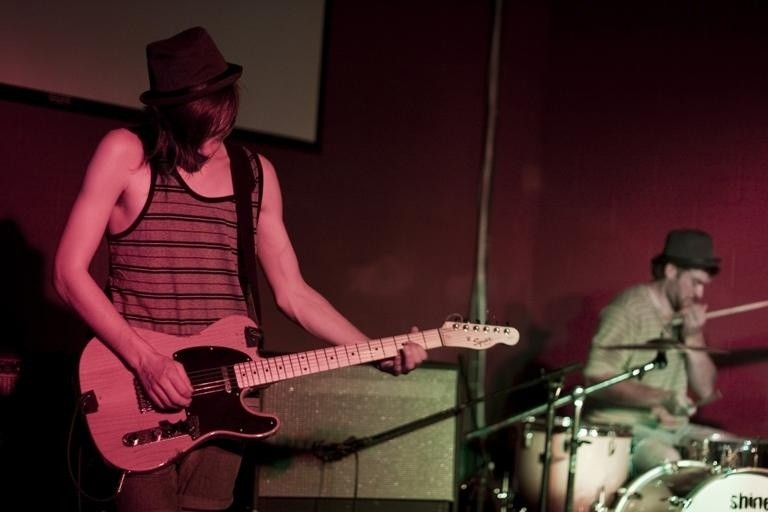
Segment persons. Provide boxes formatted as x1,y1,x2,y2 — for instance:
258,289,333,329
582,228,747,471
51,24,429,511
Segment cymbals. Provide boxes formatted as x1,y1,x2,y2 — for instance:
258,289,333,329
598,337,726,353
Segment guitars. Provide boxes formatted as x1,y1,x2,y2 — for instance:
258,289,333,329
79,314,521,476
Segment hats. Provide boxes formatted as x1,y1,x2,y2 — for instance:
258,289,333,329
656,229,722,267
139,26,243,106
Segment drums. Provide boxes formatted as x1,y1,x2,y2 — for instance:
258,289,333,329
702,438,768,467
517,418,635,511
611,457,768,512
